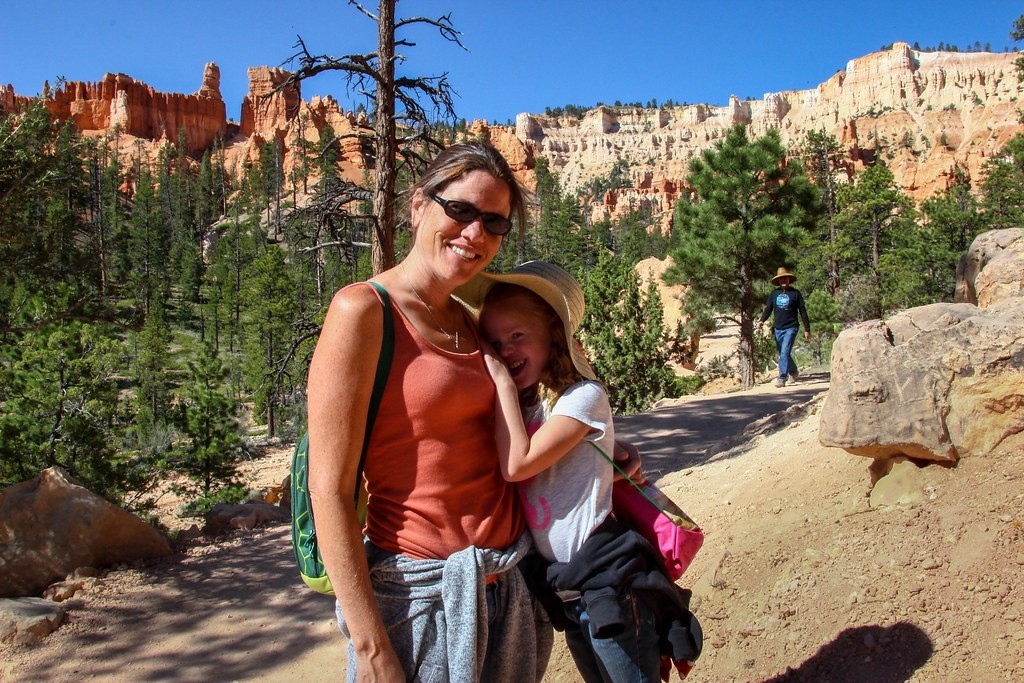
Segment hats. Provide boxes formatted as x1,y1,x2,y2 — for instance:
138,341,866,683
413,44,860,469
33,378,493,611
770,267,797,286
450,260,596,381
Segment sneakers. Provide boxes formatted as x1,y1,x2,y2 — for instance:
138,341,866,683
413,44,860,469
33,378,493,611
774,372,800,388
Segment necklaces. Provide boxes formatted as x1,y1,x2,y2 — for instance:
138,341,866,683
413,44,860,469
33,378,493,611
402,263,460,350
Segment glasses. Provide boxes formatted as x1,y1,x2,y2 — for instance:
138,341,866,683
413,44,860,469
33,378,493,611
427,192,512,235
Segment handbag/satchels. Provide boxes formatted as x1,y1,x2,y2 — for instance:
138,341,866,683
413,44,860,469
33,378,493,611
580,441,703,582
291,282,394,595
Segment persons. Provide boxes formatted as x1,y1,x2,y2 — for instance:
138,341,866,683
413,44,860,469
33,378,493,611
307,140,557,682
451,260,667,682
756,267,810,387
